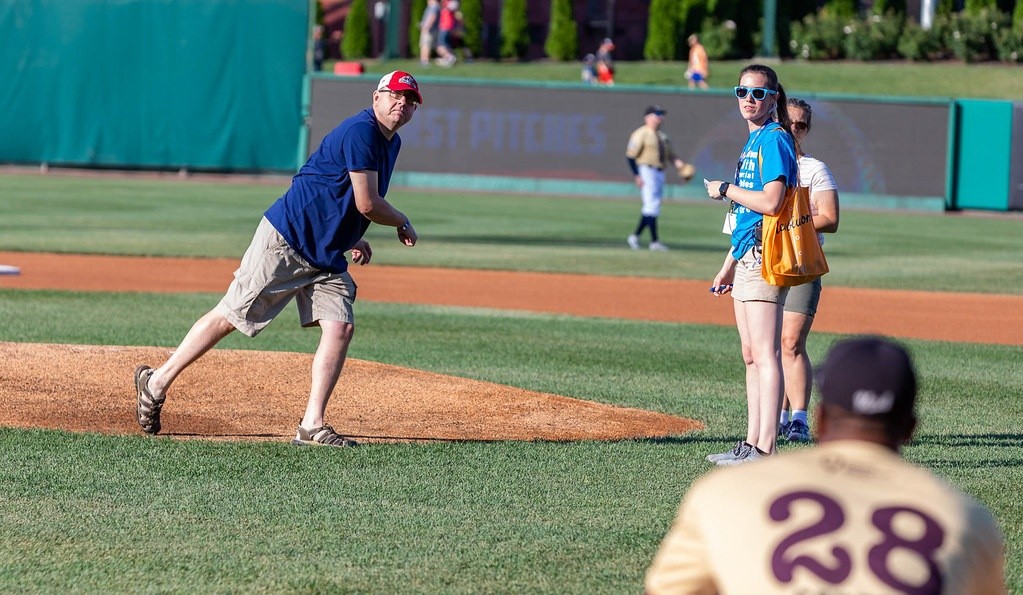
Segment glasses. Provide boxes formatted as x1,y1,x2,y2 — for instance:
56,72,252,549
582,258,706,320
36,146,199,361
734,86,777,100
791,121,808,129
379,89,418,105
651,111,664,116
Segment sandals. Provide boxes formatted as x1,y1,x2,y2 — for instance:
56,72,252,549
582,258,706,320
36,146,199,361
291,418,358,448
134,365,165,433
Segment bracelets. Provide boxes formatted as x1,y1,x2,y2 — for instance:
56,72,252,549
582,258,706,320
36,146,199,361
718,181,729,196
398,217,410,233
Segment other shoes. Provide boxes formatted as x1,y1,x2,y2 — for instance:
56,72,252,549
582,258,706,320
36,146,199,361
626,233,641,250
785,419,809,442
649,241,669,252
775,421,791,440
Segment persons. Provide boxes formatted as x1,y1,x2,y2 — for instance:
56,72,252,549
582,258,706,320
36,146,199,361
134,66,423,450
625,103,684,250
638,334,1008,595
580,37,616,86
707,63,839,467
416,0,461,66
686,34,709,88
313,23,327,70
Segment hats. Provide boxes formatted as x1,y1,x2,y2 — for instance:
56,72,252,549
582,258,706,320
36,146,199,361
377,70,423,104
643,105,665,115
816,340,914,417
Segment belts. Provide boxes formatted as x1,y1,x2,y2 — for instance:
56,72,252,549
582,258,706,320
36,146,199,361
648,164,663,172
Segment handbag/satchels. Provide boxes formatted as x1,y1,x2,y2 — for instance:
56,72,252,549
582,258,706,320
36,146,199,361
758,127,829,286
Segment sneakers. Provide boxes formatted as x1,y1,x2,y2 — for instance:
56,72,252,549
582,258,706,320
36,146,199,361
705,441,752,462
716,446,777,465
